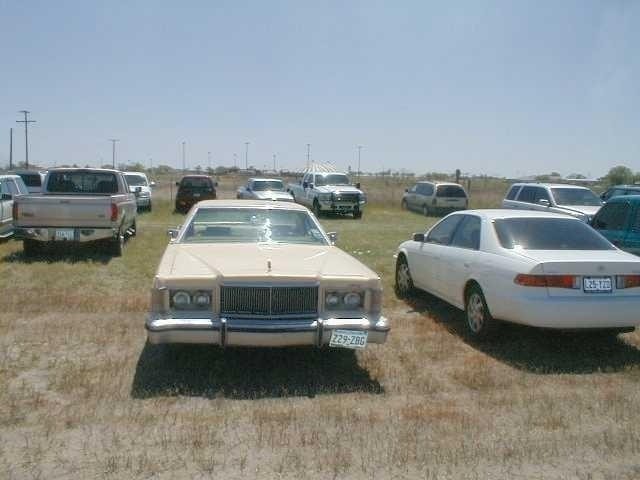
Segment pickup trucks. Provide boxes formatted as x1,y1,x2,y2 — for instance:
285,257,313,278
0,166,158,261
285,171,367,220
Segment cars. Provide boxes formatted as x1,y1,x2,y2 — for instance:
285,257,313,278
146,199,392,359
597,183,640,203
236,178,296,206
399,181,471,217
393,208,640,336
172,175,220,215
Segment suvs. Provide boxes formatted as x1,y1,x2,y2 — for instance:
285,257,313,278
501,182,606,226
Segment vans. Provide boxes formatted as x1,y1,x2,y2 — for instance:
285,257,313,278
583,196,640,256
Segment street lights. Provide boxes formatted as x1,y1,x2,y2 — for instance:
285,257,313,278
108,138,122,168
175,137,373,183
